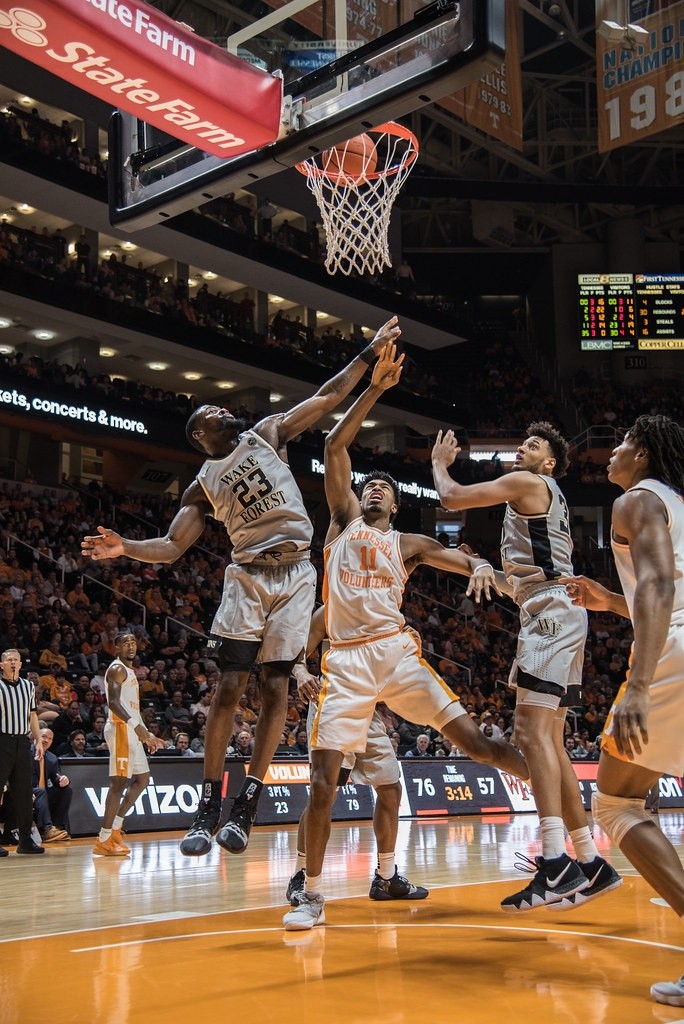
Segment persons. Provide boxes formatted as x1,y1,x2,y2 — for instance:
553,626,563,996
0,649,45,857
558,414,684,1007
282,339,533,931
82,316,401,857
0,353,634,761
431,423,623,912
92,632,158,856
0,108,684,448
286,604,429,907
0,728,72,846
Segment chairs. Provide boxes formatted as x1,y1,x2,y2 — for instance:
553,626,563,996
18,579,308,758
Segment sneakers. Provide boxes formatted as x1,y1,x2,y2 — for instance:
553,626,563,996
369,864,429,900
180,797,225,855
283,891,325,930
651,976,684,1006
286,869,306,905
216,795,258,853
541,856,621,912
93,834,126,856
501,852,589,911
111,829,128,849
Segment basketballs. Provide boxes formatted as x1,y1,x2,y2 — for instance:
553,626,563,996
322,133,378,188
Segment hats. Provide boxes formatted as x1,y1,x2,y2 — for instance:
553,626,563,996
469,713,478,718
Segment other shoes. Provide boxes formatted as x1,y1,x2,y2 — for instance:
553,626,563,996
0,847,9,857
42,826,70,842
0,829,18,845
16,841,45,854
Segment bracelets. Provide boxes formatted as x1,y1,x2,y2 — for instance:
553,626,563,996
359,345,376,365
127,718,140,729
474,564,493,573
